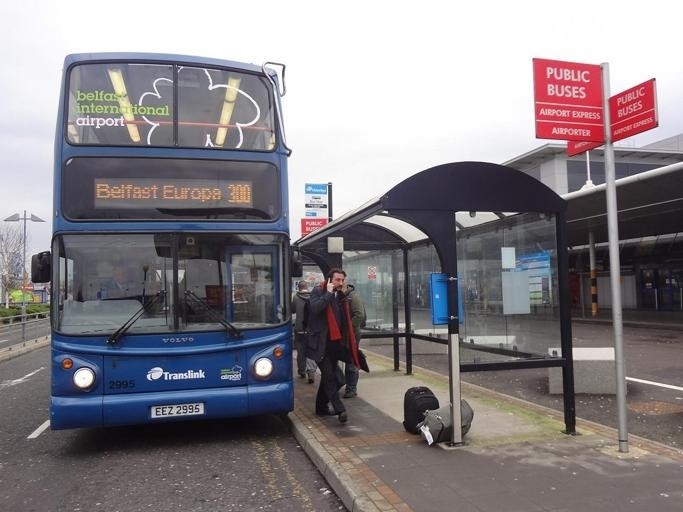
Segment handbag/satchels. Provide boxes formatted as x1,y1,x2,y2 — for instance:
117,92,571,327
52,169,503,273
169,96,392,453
416,399,475,443
303,330,321,351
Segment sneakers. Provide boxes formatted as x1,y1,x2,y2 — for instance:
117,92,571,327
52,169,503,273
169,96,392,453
344,391,355,397
298,369,314,382
316,409,347,422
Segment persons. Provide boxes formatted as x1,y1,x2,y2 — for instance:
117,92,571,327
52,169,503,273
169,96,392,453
97,266,135,299
291,281,318,383
414,281,421,308
304,267,360,422
342,281,367,398
643,271,656,306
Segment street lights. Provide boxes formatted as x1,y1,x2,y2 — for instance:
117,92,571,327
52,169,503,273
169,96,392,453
4,211,45,341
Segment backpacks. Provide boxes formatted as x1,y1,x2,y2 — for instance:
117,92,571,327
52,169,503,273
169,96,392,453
403,386,440,435
296,294,310,329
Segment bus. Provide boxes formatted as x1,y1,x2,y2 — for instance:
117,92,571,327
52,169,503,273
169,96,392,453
30,54,302,431
